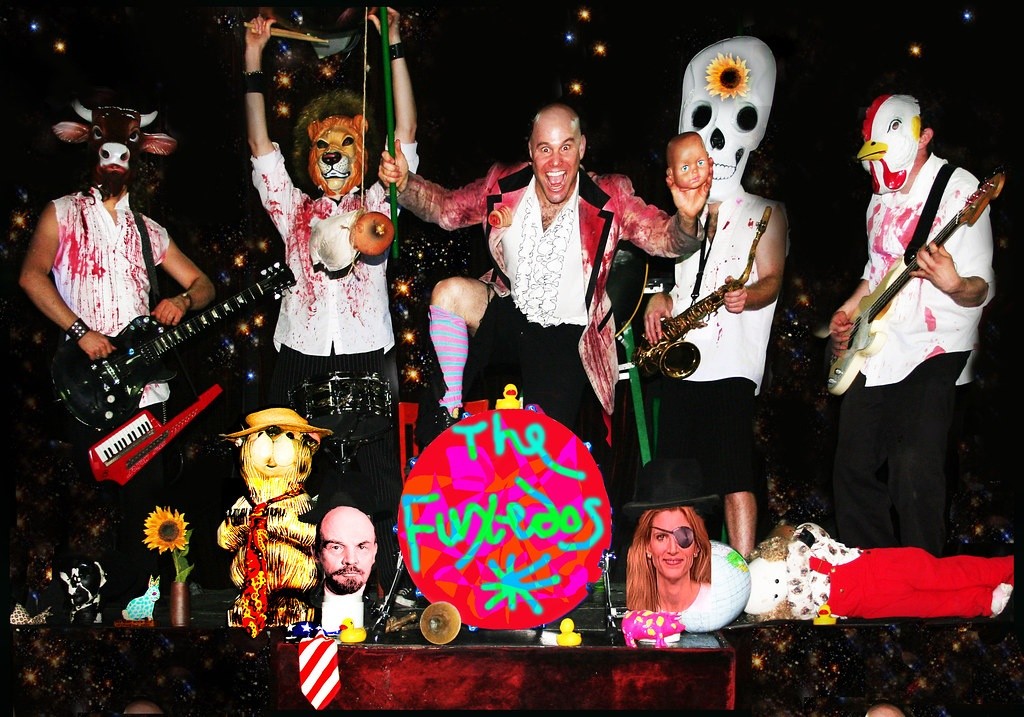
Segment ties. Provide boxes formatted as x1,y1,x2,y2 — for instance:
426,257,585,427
244,482,308,637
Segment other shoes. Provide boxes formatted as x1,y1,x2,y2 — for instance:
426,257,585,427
425,406,465,446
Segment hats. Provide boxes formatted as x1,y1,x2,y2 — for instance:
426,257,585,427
218,403,334,440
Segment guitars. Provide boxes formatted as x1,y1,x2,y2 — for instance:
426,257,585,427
827,169,1007,397
49,261,296,435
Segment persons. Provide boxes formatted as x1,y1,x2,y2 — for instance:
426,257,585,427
626,504,711,616
243,6,420,607
643,34,789,562
829,86,997,557
296,506,380,640
665,131,710,189
377,103,714,473
20,84,217,361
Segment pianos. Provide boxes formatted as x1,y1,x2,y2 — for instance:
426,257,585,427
86,382,222,487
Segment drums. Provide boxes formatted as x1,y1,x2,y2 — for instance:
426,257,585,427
283,370,396,447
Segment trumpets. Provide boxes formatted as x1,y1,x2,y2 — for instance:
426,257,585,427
385,601,462,645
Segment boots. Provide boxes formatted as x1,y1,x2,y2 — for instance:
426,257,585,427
69,567,100,624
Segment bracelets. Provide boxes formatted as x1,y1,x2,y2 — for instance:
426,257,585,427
245,70,264,93
181,291,193,311
389,42,405,61
66,318,90,343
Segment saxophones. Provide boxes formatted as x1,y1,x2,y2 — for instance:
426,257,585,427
634,204,773,381
